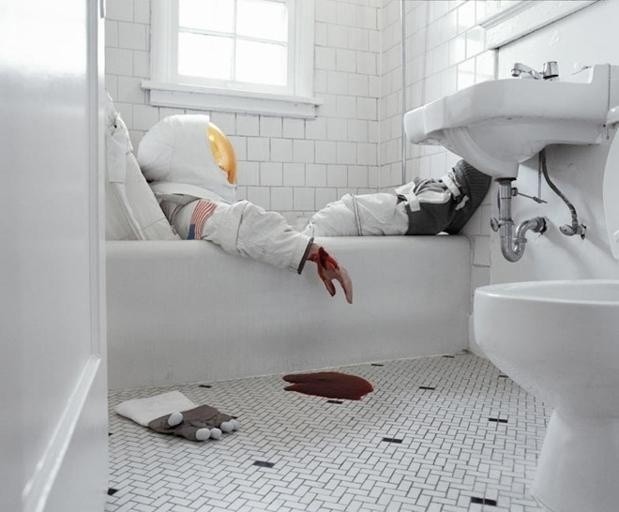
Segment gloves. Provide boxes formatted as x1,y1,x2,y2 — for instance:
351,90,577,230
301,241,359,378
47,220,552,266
114,390,240,441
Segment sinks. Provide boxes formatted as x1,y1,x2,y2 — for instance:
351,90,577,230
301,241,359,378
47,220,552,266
401,63,612,180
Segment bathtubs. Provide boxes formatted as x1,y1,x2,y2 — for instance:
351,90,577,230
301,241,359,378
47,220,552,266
107,234,473,391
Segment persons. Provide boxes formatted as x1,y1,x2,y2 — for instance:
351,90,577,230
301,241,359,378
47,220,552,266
139,114,493,305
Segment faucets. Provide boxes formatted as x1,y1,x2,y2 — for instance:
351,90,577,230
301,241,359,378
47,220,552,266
511,59,559,80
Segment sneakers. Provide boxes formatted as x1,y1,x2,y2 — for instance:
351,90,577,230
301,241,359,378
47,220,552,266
442,160,492,235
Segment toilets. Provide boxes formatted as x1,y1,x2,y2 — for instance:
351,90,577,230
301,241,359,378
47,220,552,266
469,105,619,511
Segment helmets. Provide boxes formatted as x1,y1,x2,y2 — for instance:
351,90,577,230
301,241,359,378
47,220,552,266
137,114,237,205
